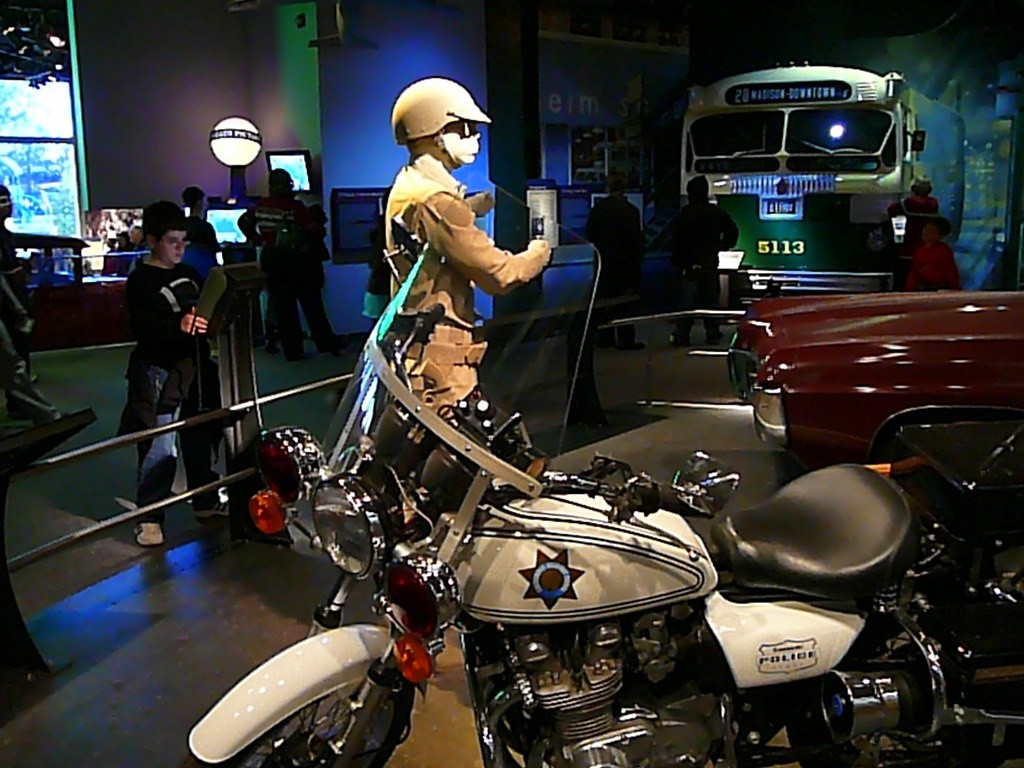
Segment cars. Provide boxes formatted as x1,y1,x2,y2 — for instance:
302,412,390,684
724,289,1022,548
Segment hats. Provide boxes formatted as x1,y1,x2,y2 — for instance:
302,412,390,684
686,176,708,198
606,173,622,188
182,186,204,208
269,169,293,186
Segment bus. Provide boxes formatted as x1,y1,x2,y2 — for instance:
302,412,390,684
681,61,926,295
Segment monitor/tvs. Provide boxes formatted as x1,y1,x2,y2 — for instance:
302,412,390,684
265,148,314,194
207,209,248,245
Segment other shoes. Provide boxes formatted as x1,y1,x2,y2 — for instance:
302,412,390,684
706,327,723,343
316,335,349,353
192,500,229,517
136,522,164,545
264,345,279,355
597,339,616,349
618,343,646,351
283,346,305,361
669,331,690,346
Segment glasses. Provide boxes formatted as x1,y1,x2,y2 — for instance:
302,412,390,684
160,235,191,246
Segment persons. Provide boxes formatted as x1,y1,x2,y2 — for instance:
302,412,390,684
885,178,960,293
670,175,737,346
386,78,553,500
584,171,648,351
184,170,337,362
114,199,230,547
0,184,61,421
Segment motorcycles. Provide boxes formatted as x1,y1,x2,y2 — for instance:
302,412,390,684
177,239,1024,768
